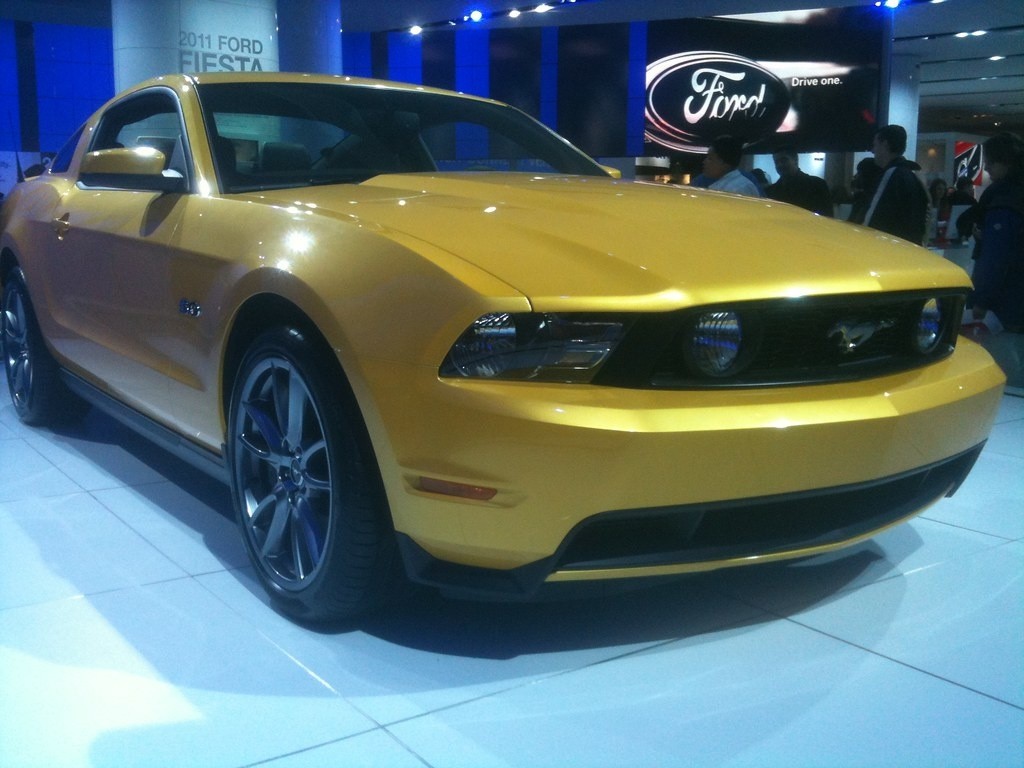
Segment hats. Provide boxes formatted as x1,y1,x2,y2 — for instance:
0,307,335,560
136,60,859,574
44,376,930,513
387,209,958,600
712,135,742,166
956,175,974,187
857,157,877,173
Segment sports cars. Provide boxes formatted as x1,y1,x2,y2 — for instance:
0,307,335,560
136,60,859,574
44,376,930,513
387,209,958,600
0,70,1006,637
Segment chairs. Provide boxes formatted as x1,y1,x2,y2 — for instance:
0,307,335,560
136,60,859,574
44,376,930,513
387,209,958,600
171,133,238,191
260,142,313,173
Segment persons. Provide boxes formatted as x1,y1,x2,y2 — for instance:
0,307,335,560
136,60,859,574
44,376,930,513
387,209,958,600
848,124,932,247
766,146,834,218
948,176,977,206
702,134,758,197
929,178,946,209
948,187,955,193
970,131,1024,332
692,168,768,196
